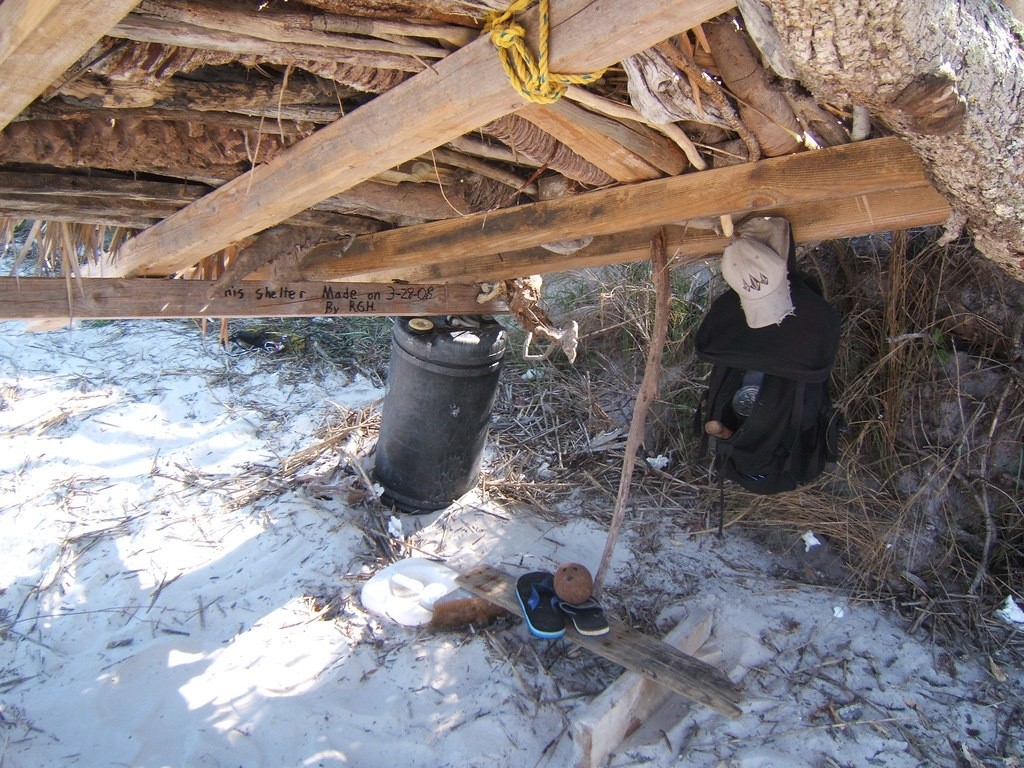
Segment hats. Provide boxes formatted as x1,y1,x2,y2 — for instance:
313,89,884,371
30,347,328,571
721,215,797,329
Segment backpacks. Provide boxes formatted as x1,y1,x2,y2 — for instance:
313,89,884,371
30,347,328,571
692,222,842,495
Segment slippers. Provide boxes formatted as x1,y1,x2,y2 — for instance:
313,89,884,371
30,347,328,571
560,596,609,637
515,571,566,640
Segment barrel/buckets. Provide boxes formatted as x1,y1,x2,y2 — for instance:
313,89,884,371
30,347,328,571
373,317,507,515
